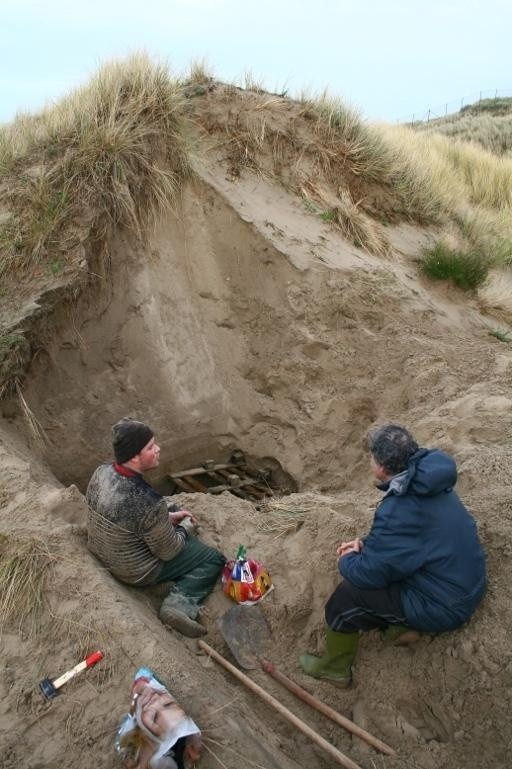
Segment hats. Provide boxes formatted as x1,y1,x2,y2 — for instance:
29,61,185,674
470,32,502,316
110,417,154,466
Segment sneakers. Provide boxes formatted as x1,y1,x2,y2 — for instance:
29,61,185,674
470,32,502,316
157,595,208,638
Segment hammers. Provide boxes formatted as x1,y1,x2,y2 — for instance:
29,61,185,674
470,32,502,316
38,651,104,699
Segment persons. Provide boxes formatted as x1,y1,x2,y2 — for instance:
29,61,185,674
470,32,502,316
297,422,486,689
85,417,227,638
128,674,188,742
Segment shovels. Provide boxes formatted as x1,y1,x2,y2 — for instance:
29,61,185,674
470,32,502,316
219,605,397,757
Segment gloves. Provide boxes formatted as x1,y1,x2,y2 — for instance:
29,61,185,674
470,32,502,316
176,515,203,537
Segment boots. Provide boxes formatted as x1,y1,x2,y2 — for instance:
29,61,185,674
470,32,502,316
299,623,359,689
381,624,419,646
